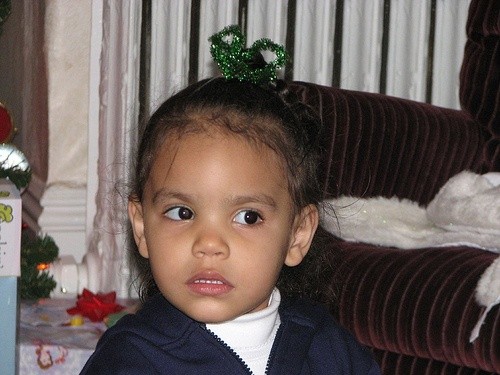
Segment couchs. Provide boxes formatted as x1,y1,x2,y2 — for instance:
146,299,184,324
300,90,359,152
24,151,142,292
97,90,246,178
275,0,500,375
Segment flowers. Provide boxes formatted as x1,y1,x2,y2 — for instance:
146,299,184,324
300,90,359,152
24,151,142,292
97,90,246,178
66,288,126,322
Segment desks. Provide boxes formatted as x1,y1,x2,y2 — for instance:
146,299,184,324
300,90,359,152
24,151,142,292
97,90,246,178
18,297,141,375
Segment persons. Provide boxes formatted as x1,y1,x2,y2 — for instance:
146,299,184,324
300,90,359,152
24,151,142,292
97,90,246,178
78,47,384,375
